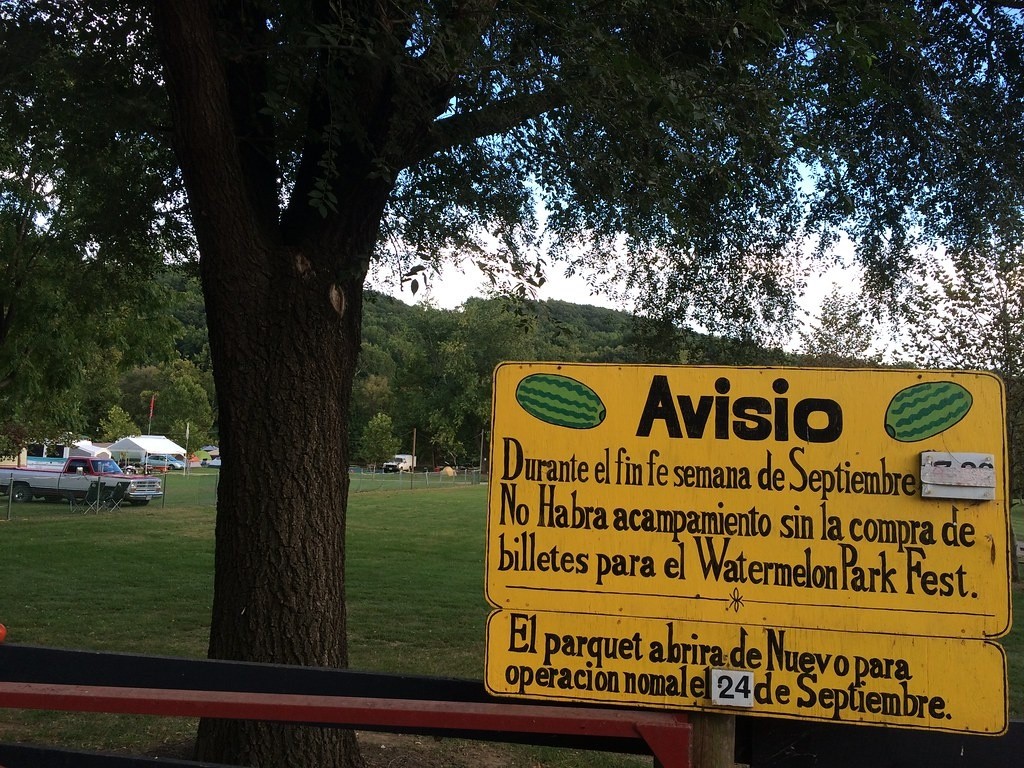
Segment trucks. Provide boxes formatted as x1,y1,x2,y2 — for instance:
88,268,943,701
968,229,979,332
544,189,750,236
383,454,417,473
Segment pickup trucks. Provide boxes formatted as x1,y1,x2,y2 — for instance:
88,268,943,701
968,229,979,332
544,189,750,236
0,455,164,507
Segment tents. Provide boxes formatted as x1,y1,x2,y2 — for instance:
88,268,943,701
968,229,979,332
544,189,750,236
440,466,457,477
107,437,187,476
189,445,222,469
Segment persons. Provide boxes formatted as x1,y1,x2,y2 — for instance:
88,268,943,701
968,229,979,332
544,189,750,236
117,459,146,469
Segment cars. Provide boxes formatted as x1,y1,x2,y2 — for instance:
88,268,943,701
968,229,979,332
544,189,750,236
144,453,186,471
